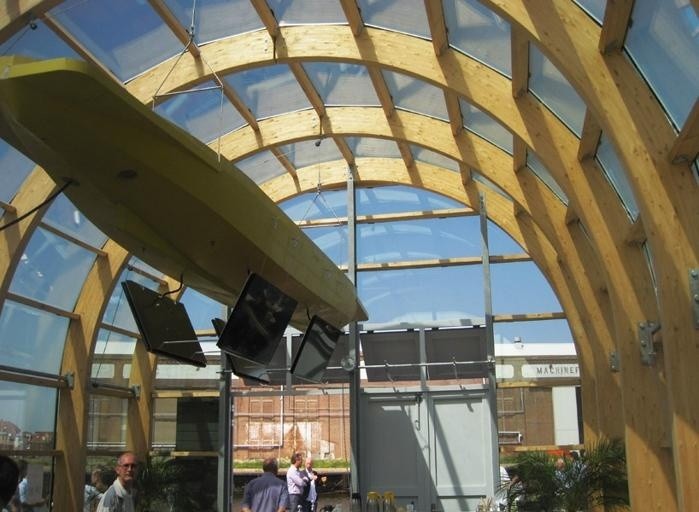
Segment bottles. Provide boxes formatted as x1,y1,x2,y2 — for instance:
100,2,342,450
349,491,395,511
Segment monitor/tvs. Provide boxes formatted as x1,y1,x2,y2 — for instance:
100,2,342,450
290,313,342,384
120,279,208,368
210,318,271,385
216,271,298,367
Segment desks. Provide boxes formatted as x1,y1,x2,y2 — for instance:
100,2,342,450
332,500,417,512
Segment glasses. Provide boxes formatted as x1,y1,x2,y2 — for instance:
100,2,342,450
119,464,138,468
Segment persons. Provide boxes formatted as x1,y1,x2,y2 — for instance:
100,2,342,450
507,474,520,490
0,453,116,512
301,458,321,511
96,450,140,511
240,457,290,512
286,452,310,512
567,450,587,484
555,458,569,492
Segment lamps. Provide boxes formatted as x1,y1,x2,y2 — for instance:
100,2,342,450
341,356,356,372
